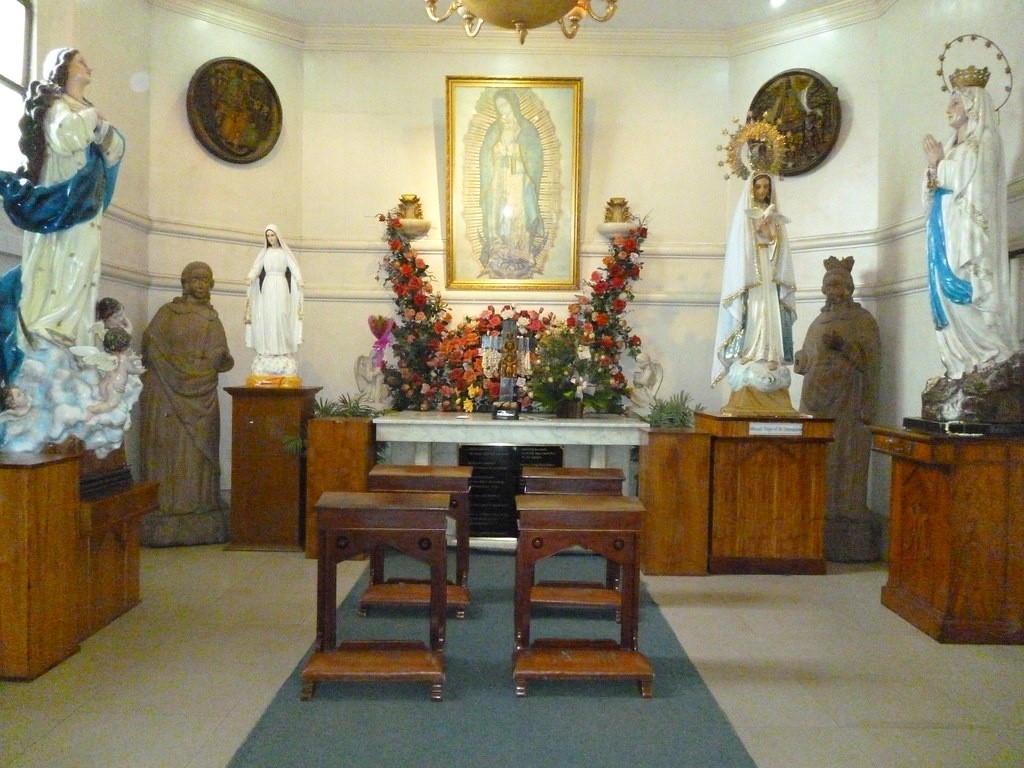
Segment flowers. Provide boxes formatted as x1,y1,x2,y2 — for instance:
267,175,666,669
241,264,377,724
368,209,648,418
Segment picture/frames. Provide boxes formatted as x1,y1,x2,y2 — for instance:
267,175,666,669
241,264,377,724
444,74,582,292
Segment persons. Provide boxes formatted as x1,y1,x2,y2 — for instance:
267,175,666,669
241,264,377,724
733,168,786,372
502,331,517,352
142,260,233,516
362,347,389,403
20,41,122,351
922,81,1018,379
0,384,41,425
793,267,881,523
245,224,305,355
86,325,146,424
502,356,517,377
501,343,518,377
90,296,133,351
628,353,657,408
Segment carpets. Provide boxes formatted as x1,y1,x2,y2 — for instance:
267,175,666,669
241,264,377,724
223,546,760,768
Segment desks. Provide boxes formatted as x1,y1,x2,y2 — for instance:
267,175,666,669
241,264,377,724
510,493,657,699
358,463,474,619
521,465,627,624
299,490,454,701
372,409,651,554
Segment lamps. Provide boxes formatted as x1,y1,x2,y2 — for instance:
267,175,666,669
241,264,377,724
425,0,617,45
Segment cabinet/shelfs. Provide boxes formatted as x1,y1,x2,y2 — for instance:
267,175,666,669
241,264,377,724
304,416,376,559
867,425,1022,645
637,427,713,578
223,386,322,552
0,439,160,680
692,408,837,576
